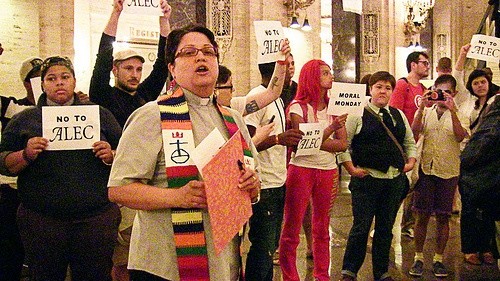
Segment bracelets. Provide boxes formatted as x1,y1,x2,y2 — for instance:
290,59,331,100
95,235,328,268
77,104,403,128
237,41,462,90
274,134,280,145
102,150,116,167
251,187,261,205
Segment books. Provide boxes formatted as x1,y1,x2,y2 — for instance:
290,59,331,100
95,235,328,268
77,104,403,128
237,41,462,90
200,127,254,258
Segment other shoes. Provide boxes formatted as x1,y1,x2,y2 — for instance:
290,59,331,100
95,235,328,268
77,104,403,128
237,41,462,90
271,248,280,264
306,247,314,258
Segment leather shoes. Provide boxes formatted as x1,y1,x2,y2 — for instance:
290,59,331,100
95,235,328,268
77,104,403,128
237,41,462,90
462,250,494,266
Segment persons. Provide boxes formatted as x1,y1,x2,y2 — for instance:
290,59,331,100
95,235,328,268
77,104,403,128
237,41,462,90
241,62,305,281
1,41,91,281
209,49,300,151
408,72,472,277
454,68,498,266
88,0,172,281
1,53,123,281
277,58,349,281
334,71,419,281
455,95,500,221
106,22,264,281
344,42,500,159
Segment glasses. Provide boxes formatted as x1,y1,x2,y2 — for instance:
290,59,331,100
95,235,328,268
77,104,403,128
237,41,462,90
171,47,219,64
216,82,234,93
414,59,431,67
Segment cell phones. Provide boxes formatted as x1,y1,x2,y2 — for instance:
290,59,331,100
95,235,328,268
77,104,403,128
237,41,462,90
268,115,275,124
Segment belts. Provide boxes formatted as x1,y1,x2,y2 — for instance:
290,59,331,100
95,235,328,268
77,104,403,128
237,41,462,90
21,193,117,222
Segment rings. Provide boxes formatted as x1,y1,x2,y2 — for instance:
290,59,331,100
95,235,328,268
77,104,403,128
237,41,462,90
251,176,256,182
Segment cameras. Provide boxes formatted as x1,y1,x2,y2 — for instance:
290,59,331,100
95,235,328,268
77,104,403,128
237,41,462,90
427,91,445,100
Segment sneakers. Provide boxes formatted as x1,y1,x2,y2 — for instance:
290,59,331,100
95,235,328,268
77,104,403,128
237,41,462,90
400,226,414,238
408,260,449,276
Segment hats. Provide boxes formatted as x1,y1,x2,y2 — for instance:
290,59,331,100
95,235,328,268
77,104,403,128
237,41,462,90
41,55,74,91
113,50,145,65
19,56,44,82
